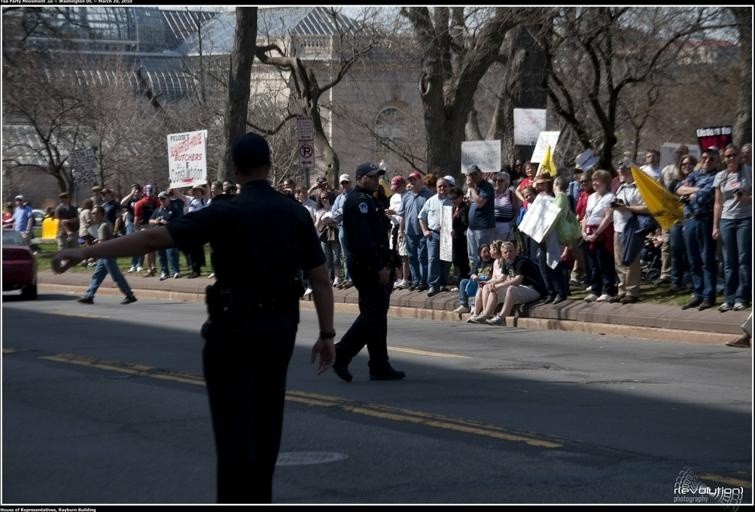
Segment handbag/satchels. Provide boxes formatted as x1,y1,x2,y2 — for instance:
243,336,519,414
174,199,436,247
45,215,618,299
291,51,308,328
557,212,583,245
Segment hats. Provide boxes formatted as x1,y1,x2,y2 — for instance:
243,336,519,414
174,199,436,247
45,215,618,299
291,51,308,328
465,164,480,176
339,173,352,184
390,175,405,191
59,185,206,199
407,172,421,180
15,195,24,201
356,162,386,179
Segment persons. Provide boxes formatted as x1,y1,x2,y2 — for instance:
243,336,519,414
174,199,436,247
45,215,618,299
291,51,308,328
327,162,409,384
2,143,753,350
53,135,339,504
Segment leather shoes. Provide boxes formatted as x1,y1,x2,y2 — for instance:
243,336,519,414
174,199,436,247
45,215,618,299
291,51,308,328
725,336,750,348
686,299,701,308
654,278,672,286
677,289,692,296
662,287,688,297
544,277,638,304
699,300,716,310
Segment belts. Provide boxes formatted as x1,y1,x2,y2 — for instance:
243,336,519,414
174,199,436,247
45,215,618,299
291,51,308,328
688,213,700,220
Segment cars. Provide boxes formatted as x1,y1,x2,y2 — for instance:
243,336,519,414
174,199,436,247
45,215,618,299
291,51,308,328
31,209,46,226
3,229,40,301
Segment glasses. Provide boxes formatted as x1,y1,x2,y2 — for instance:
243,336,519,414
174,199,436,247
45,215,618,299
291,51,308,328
580,179,590,184
681,151,739,167
449,195,459,201
495,178,504,183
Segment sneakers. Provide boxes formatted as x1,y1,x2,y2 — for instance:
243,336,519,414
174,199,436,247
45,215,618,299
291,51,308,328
333,357,353,383
734,303,746,311
453,305,505,326
333,278,352,289
79,298,93,304
121,295,137,304
128,266,201,280
369,363,405,380
392,278,458,296
718,302,732,311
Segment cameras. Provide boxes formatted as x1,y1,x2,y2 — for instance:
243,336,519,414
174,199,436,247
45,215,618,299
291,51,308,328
732,188,743,198
679,195,689,204
610,197,624,208
